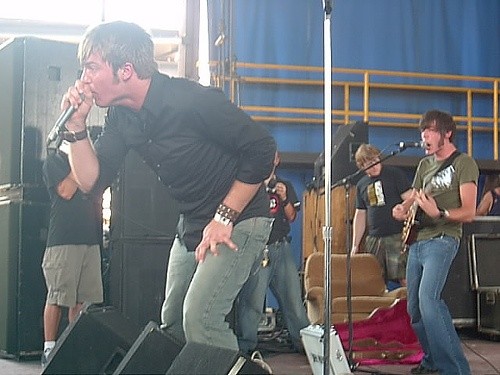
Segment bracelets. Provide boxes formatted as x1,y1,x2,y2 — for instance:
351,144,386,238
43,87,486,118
438,208,449,221
281,199,289,205
214,204,240,226
62,129,88,142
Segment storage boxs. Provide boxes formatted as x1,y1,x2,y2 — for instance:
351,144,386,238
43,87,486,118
452,215,500,336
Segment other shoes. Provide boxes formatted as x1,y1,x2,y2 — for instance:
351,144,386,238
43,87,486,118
411,364,438,375
41,347,54,367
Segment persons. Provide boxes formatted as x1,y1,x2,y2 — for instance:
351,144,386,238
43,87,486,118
476,175,500,215
41,131,103,371
351,143,408,288
392,110,478,375
60,21,274,353
236,149,313,358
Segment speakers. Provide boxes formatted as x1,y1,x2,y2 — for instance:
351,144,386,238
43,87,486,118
314,121,369,188
0,35,273,375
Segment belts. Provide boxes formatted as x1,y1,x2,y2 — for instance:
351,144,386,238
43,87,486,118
275,237,292,242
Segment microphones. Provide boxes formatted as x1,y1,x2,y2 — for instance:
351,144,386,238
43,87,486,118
396,142,428,149
45,93,86,146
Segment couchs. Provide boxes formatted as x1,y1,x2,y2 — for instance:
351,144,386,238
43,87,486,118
304,254,409,327
